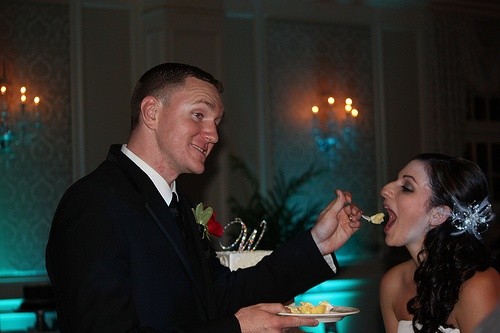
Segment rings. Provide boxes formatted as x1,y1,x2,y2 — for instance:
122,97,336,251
348,214,354,220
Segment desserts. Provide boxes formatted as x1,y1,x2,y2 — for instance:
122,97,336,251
286,301,333,314
370,212,385,225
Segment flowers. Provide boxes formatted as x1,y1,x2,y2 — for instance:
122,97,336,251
192,202,223,241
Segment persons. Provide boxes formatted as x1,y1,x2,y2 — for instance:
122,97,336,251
376,151,499,333
44,63,363,333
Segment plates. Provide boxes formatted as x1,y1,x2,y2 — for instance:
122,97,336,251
277,306,360,322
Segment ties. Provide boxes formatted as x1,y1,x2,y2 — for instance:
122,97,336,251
169,191,199,278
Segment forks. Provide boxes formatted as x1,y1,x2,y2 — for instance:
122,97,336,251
350,201,370,222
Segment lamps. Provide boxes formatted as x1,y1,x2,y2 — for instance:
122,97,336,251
312,96,359,152
0,86,41,155
